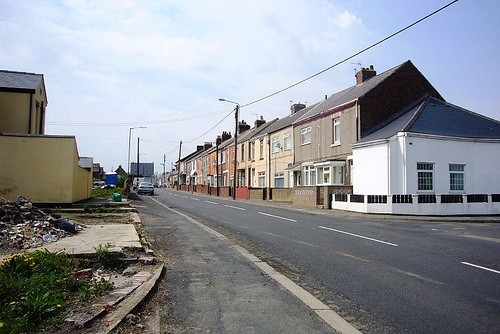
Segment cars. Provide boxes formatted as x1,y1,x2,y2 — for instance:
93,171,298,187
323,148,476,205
137,182,154,195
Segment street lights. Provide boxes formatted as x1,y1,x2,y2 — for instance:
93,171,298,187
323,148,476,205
219,98,240,199
126,126,147,182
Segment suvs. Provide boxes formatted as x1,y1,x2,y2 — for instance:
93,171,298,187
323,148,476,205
133,178,145,190
